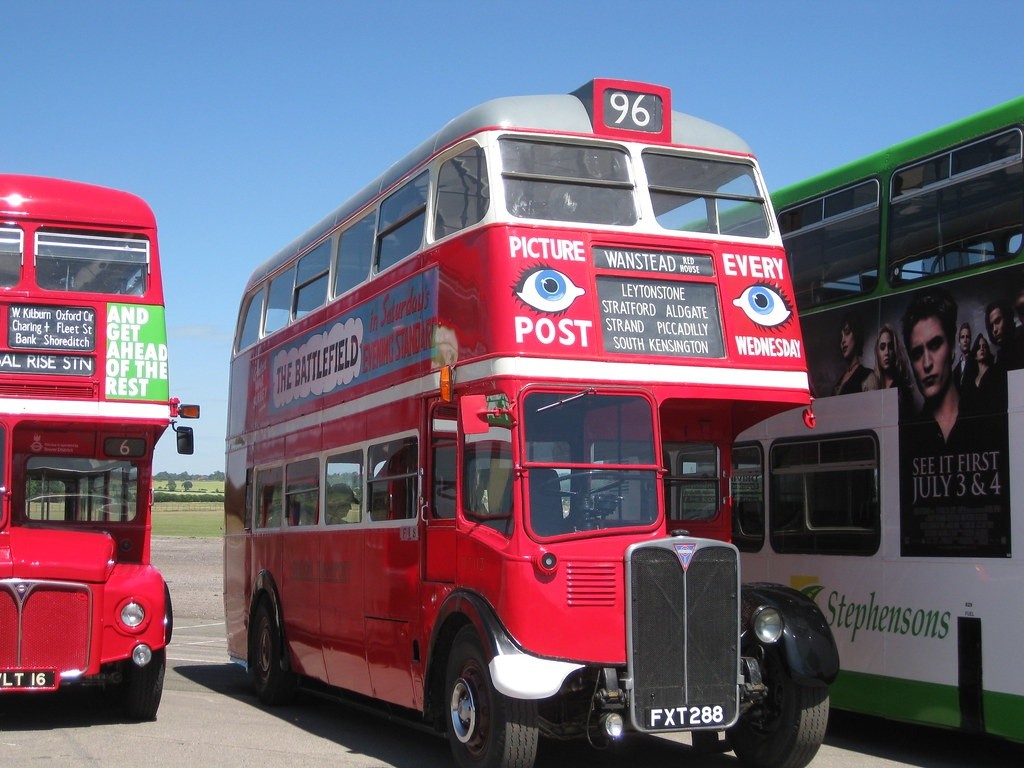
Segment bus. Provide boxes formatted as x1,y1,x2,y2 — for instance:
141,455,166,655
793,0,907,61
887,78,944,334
227,77,835,768
0,172,203,726
582,92,1024,759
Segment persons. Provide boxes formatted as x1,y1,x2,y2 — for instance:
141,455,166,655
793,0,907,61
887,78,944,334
861,322,914,419
899,289,1010,507
952,323,979,398
325,483,359,525
985,300,1024,412
968,333,994,393
832,311,878,396
1015,288,1024,345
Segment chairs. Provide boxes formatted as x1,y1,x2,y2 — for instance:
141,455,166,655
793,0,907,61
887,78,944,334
501,468,564,536
